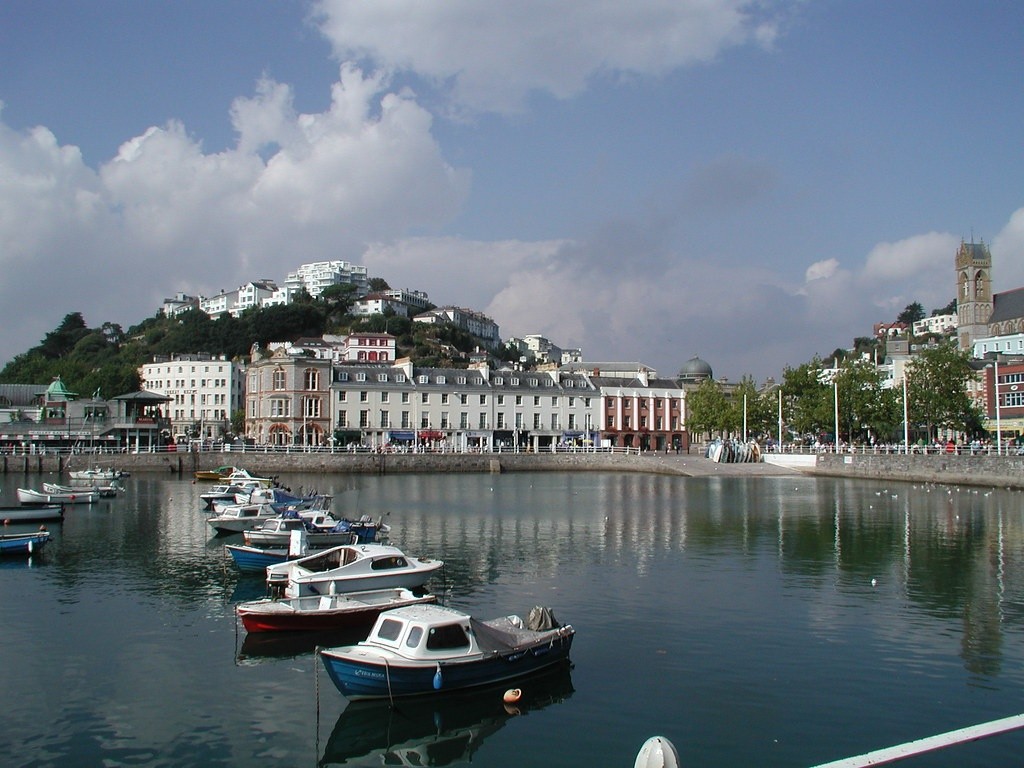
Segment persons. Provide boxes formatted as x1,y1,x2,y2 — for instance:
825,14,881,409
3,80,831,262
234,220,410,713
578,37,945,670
668,442,671,451
970,438,1015,455
675,438,680,454
913,437,962,454
839,435,905,453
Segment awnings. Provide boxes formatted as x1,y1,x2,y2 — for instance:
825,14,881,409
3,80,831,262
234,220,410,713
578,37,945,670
391,434,415,440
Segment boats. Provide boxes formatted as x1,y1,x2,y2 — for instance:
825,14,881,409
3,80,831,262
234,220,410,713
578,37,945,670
234,588,436,644
200,484,246,503
299,509,389,542
207,501,293,535
235,489,331,509
17,488,99,503
0,531,50,551
228,542,330,573
69,468,122,479
194,467,233,479
315,601,574,705
219,471,273,486
0,505,63,521
244,517,354,547
267,544,443,598
238,481,269,493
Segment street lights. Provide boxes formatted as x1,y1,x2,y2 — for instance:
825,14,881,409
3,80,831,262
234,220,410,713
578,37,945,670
299,395,306,451
513,427,522,456
586,411,588,453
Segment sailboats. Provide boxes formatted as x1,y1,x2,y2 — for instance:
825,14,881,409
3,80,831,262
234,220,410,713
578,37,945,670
42,390,116,498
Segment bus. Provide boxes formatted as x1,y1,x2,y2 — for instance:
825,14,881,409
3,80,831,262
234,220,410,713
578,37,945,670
980,418,1024,441
820,430,861,444
